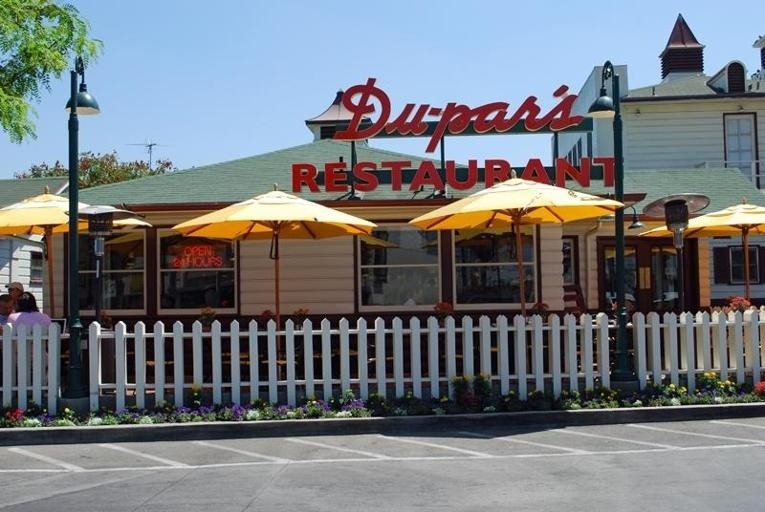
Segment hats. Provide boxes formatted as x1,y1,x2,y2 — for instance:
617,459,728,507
5,282,23,291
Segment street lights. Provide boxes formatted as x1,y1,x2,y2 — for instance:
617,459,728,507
62,56,101,394
585,61,638,382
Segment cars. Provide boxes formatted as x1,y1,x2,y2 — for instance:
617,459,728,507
170,266,237,308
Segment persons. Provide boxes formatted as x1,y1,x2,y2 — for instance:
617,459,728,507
0,294,18,335
5,291,52,396
5,282,23,312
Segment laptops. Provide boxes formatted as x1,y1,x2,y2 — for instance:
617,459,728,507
50,318,70,337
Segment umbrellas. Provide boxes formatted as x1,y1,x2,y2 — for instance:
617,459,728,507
637,194,765,307
407,167,627,319
417,220,540,264
359,230,399,254
0,185,154,318
168,182,380,355
